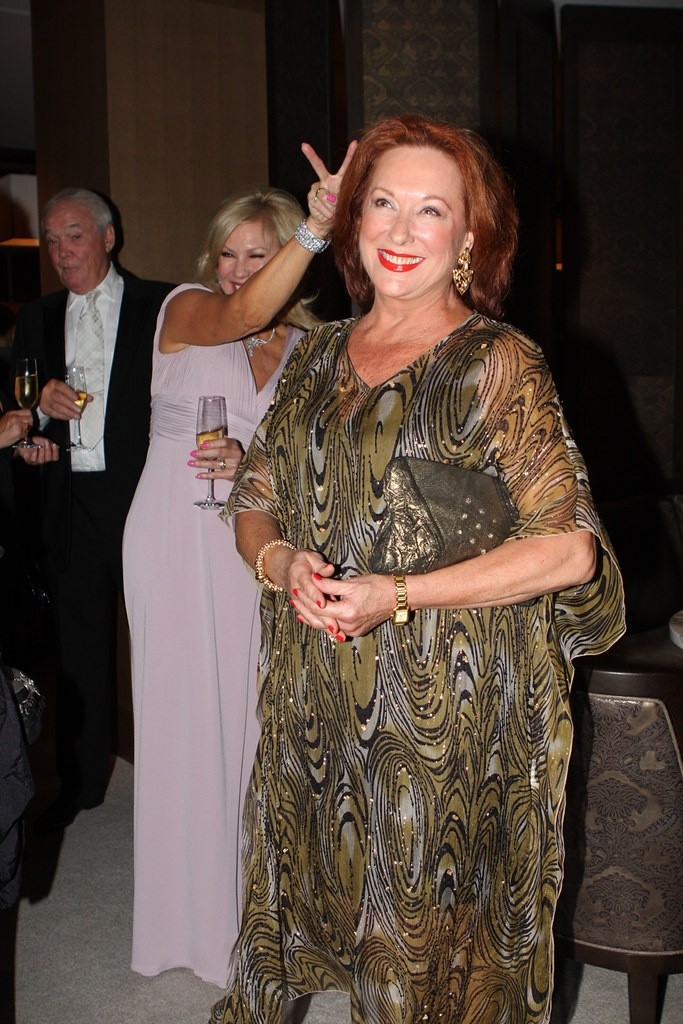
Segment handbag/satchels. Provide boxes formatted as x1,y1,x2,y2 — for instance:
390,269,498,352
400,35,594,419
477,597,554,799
370,456,541,607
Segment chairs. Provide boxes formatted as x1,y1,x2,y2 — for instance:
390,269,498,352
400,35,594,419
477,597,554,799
553,495,683,1024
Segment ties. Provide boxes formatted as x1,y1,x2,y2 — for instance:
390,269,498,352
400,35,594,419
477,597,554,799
72,290,106,449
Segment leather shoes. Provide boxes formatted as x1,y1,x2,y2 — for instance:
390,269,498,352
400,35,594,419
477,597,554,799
33,794,106,833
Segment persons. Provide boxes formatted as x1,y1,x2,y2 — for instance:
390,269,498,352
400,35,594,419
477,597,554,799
119,138,356,989
201,116,630,1022
0,187,173,916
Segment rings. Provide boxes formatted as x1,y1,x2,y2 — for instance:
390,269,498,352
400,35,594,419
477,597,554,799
328,636,339,644
315,187,326,198
219,458,226,470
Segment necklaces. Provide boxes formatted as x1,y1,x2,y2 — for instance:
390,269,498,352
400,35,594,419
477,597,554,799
246,327,276,358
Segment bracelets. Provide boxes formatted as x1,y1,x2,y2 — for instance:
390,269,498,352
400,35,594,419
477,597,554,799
254,539,297,592
294,219,331,253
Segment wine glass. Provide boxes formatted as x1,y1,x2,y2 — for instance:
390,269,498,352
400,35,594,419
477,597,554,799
193,396,228,510
12,358,42,448
63,366,92,451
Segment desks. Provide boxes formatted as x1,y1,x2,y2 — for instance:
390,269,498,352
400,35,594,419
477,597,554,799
604,607,683,674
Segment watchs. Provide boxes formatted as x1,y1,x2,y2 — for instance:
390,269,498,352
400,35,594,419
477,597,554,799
392,570,409,628
